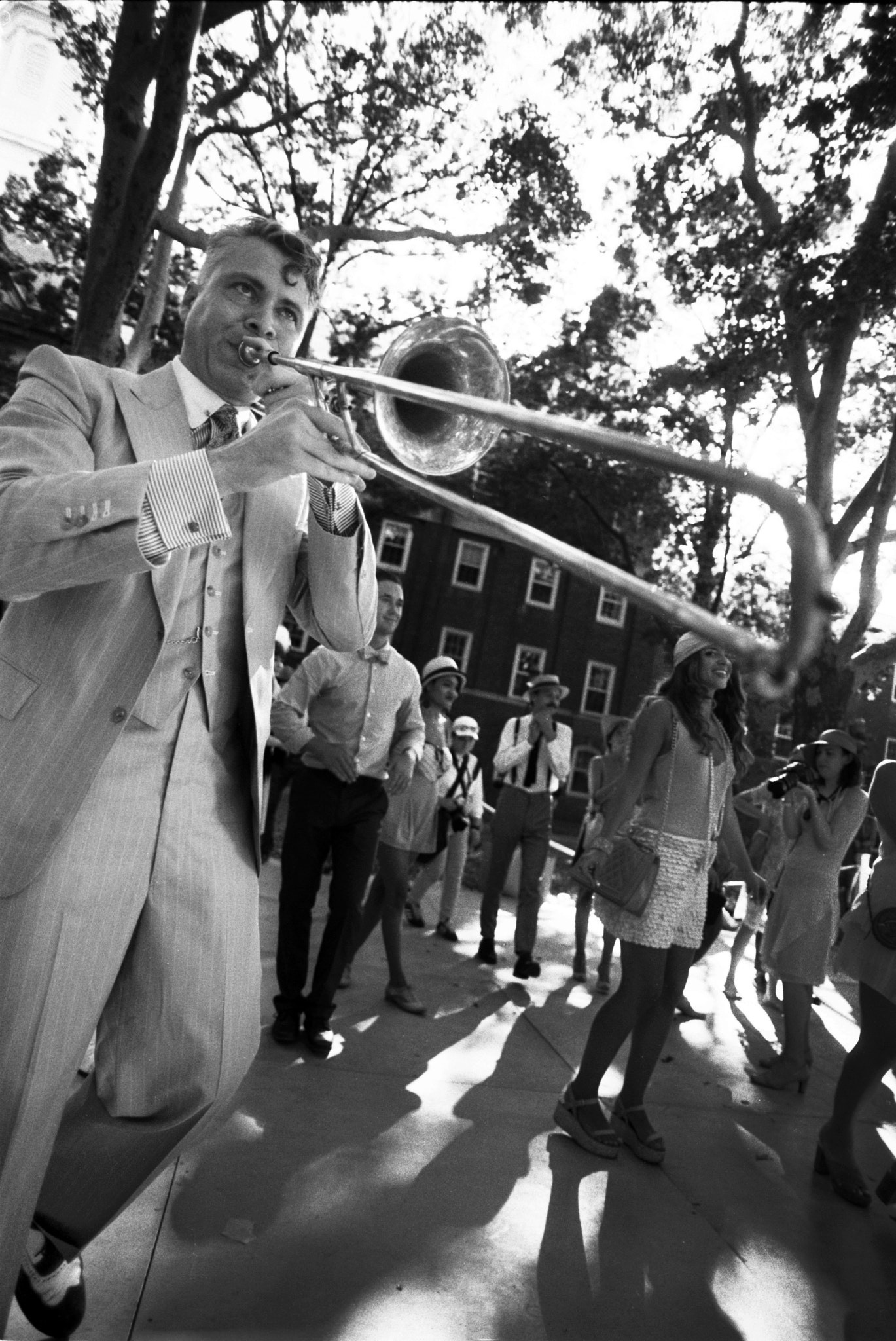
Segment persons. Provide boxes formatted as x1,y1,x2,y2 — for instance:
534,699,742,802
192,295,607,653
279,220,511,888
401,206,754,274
554,632,775,1163
404,715,483,942
270,572,426,1054
478,675,572,978
814,759,896,1207
339,657,467,1013
0,215,379,1341
743,728,870,1094
274,640,285,697
724,744,807,1011
572,715,631,996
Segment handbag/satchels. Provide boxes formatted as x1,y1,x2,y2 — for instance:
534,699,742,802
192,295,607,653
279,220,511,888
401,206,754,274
570,835,660,916
873,907,896,950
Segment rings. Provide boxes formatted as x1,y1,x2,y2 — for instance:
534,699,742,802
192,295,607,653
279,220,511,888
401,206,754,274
546,718,548,721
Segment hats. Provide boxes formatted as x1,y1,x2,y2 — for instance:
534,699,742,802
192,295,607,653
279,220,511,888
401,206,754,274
674,630,714,666
523,674,571,703
451,716,480,741
422,656,466,692
805,728,857,766
600,714,626,738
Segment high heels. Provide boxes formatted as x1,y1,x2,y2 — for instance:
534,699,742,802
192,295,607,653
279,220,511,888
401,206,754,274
610,1094,666,1163
753,1063,809,1093
814,1137,872,1207
759,1050,814,1068
554,1082,624,1158
875,1158,896,1206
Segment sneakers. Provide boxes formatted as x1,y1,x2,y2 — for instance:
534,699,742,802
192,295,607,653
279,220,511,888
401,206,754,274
15,1219,86,1336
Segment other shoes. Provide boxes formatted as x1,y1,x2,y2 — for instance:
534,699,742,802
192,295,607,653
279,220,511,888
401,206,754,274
385,984,426,1013
306,1014,333,1051
272,1008,300,1042
338,964,351,987
405,900,820,1019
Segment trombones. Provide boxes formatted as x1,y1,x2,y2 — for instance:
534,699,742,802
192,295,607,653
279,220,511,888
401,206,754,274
235,315,843,702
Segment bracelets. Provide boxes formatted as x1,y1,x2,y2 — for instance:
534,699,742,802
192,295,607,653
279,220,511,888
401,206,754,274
588,836,614,856
782,801,793,807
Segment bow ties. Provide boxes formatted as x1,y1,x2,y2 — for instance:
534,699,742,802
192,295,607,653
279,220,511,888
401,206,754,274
360,645,392,665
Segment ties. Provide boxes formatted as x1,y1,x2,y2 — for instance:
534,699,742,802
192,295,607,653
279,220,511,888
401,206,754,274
524,731,543,787
209,405,240,451
